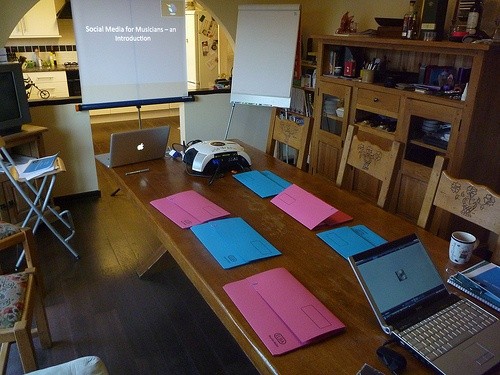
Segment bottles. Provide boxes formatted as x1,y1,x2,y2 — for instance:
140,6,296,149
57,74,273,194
465,0,483,35
35,50,57,67
401,0,418,40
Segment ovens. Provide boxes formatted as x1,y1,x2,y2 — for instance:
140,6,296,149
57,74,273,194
66,70,81,96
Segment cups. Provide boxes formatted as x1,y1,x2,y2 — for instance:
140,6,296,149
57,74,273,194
361,70,376,84
449,232,479,265
334,67,342,74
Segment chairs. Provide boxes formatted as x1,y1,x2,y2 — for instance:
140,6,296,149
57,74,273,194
0,136,80,271
266,108,314,172
336,123,405,211
417,155,500,265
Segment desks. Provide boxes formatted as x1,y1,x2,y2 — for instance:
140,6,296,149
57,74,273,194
94,139,500,375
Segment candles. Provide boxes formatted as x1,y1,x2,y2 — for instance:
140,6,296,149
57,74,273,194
466,7,479,34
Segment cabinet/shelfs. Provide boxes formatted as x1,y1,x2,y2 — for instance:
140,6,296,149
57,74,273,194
22,72,70,99
310,34,500,259
9,0,62,39
0,123,60,229
291,50,316,118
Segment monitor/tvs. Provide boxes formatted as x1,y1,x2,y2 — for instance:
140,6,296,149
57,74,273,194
0,63,32,136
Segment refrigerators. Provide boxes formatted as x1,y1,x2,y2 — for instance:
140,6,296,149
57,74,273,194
185,10,219,90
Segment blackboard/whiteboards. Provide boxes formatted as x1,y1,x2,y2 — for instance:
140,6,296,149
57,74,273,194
229,4,302,109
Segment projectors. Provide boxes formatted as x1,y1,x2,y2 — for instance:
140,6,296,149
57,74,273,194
181,140,251,175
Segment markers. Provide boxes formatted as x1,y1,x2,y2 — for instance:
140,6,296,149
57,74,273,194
232,101,272,107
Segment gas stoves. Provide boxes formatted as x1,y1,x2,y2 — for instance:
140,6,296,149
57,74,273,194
64,61,79,67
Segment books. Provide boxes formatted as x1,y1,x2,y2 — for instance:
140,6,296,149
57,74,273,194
328,51,338,74
288,52,315,117
448,260,500,312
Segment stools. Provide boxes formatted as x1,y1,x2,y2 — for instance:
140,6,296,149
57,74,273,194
0,266,53,374
0,221,46,296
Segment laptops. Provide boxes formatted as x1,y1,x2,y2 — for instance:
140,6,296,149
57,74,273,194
95,127,171,168
348,232,500,375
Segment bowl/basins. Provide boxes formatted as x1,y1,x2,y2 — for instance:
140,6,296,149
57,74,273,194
423,120,441,134
324,99,345,118
375,18,404,27
